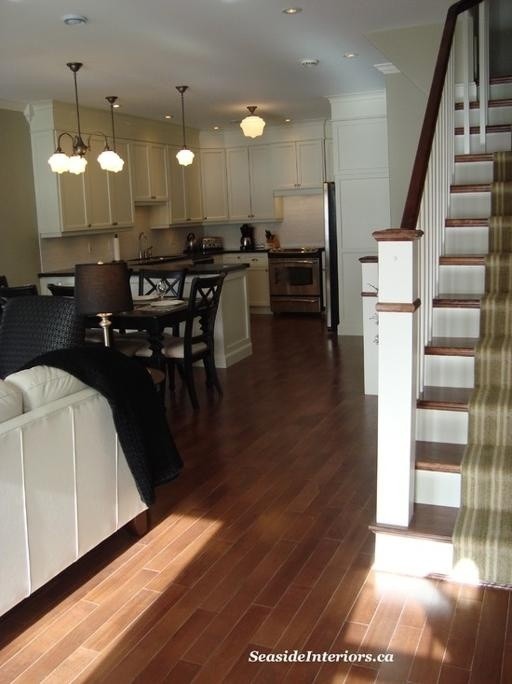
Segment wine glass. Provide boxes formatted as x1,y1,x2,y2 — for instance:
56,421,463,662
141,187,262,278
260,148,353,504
155,280,167,300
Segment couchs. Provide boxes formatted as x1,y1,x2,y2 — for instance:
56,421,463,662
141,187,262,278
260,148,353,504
0,356,167,620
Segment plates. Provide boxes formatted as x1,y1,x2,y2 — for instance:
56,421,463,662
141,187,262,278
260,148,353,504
150,300,186,305
131,295,159,301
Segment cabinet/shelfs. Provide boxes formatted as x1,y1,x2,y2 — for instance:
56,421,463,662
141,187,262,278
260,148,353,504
127,136,168,206
271,139,323,197
148,143,203,230
225,143,284,225
82,131,135,236
199,146,228,225
225,252,271,309
32,127,92,238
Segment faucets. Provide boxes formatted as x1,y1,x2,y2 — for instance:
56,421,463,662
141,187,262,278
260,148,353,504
138,232,147,258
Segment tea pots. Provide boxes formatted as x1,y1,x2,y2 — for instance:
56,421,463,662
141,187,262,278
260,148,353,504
185,231,199,251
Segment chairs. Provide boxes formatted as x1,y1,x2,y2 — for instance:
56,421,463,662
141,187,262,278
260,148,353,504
1,266,227,413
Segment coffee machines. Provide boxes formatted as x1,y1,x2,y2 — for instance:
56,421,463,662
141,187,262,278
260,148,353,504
238,222,255,252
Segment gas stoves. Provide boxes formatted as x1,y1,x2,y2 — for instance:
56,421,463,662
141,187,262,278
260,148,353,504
268,246,321,256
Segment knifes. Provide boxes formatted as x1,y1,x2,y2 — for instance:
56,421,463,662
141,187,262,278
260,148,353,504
264,229,272,240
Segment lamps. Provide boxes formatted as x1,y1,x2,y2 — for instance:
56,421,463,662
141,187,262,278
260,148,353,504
237,105,268,141
47,60,116,179
74,259,136,347
100,94,125,175
173,83,196,167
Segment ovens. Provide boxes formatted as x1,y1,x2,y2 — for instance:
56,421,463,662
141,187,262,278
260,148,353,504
269,258,321,314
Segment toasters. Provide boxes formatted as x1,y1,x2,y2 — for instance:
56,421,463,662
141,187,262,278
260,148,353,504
202,236,224,254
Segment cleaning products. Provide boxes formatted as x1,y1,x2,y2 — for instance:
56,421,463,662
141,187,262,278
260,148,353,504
113,234,121,262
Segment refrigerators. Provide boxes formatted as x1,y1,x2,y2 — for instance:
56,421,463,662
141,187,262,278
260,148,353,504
322,180,341,329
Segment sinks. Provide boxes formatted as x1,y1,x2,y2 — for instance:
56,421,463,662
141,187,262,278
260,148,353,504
129,254,186,264
